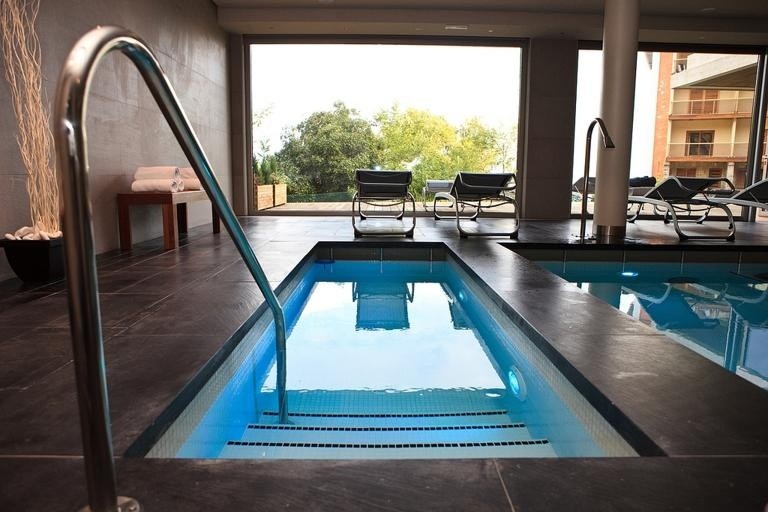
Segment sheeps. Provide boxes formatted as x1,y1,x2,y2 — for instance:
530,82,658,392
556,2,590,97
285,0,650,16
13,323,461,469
578,116,615,239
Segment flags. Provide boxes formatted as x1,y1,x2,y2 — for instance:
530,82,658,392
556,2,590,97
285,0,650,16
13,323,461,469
0,235,66,286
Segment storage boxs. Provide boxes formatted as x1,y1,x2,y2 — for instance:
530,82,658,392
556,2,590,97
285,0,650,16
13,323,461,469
117,188,220,250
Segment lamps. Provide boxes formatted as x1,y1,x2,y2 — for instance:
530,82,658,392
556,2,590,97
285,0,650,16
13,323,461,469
626,176,768,242
687,283,768,330
352,281,414,331
572,177,596,214
422,171,520,239
620,282,720,331
439,282,472,330
352,169,416,238
